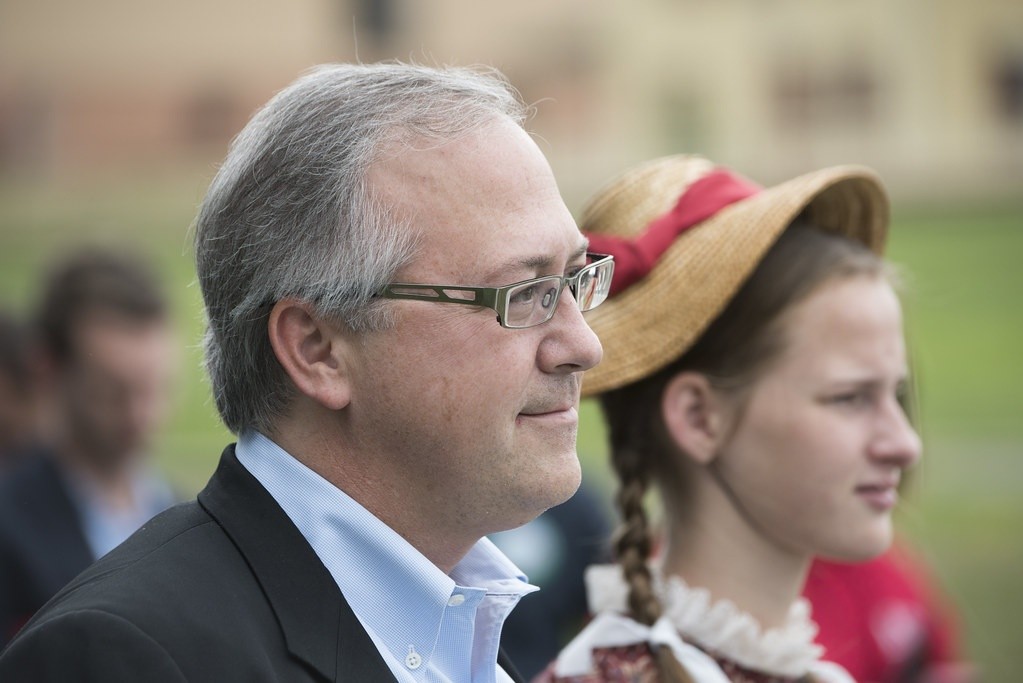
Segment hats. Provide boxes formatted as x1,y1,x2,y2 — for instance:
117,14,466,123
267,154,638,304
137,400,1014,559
577,153,888,397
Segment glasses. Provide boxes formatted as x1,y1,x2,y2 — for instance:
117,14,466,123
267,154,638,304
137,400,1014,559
315,252,615,329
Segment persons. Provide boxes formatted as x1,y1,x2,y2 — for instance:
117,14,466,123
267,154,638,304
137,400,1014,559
0,62,977,683
